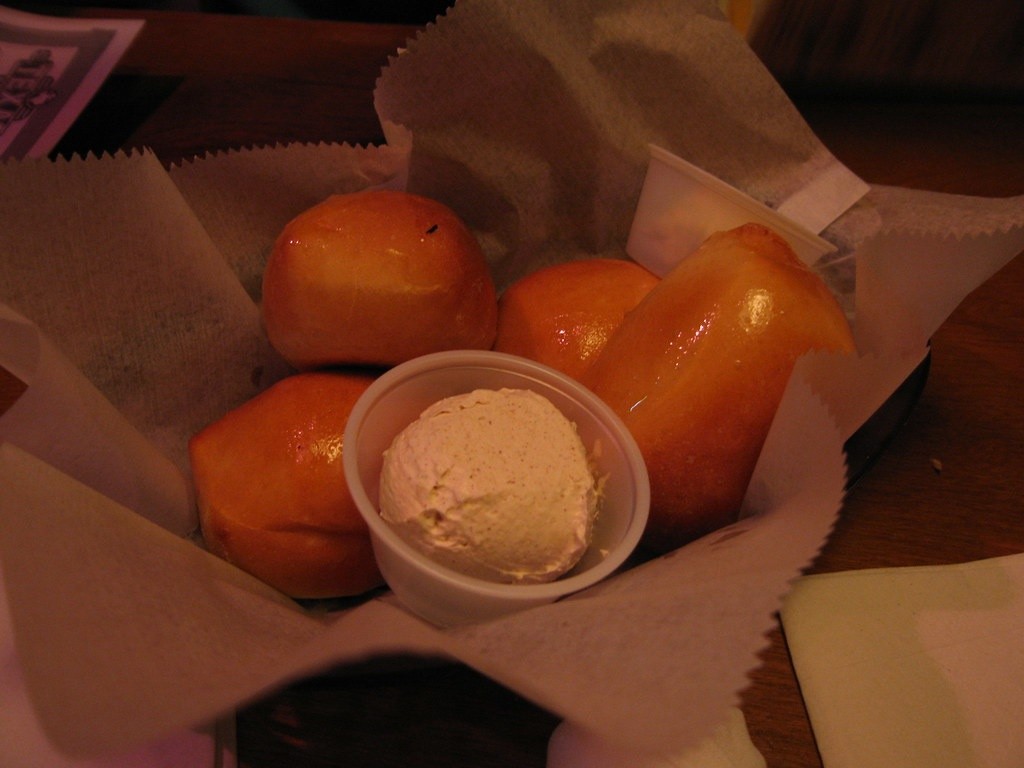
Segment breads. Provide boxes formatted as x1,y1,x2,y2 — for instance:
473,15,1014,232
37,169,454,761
188,190,862,602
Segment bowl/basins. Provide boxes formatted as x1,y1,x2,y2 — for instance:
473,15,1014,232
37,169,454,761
624,141,838,280
342,350,650,629
